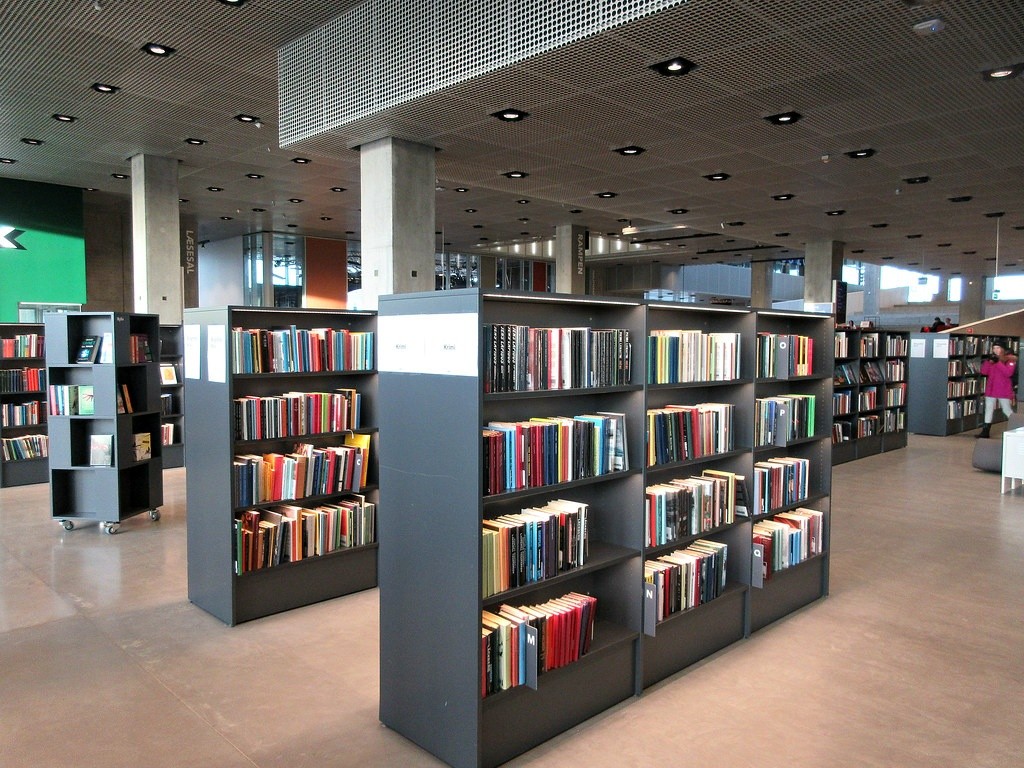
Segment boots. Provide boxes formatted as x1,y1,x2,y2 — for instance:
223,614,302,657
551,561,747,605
975,423,992,438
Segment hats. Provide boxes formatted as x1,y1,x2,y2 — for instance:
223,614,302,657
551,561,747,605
992,339,1007,351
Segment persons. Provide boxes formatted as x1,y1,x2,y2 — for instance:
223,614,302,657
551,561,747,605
847,320,856,329
932,317,951,327
975,340,1018,439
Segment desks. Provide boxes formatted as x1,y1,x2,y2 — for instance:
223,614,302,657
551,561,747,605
1002,426,1024,495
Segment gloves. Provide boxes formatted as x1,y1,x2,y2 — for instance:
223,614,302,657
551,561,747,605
982,354,999,362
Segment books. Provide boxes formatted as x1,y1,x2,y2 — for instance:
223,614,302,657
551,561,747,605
0,367,46,392
947,336,1016,419
482,591,598,698
483,325,631,393
0,401,47,427
754,332,824,580
483,412,629,494
133,433,151,461
0,332,44,358
51,384,95,416
76,333,113,365
1,434,49,461
161,394,172,415
831,332,908,444
232,325,374,577
130,334,152,363
161,423,174,445
116,384,133,414
483,499,588,598
647,330,742,621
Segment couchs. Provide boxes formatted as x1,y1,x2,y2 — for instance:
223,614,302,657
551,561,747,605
972,412,1024,472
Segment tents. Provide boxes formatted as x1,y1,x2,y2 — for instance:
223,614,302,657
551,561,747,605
90,435,112,465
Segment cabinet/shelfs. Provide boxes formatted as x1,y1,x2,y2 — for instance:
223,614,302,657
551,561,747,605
908,333,1021,437
44,312,163,534
832,329,910,466
183,305,379,627
0,324,186,489
378,288,836,768
